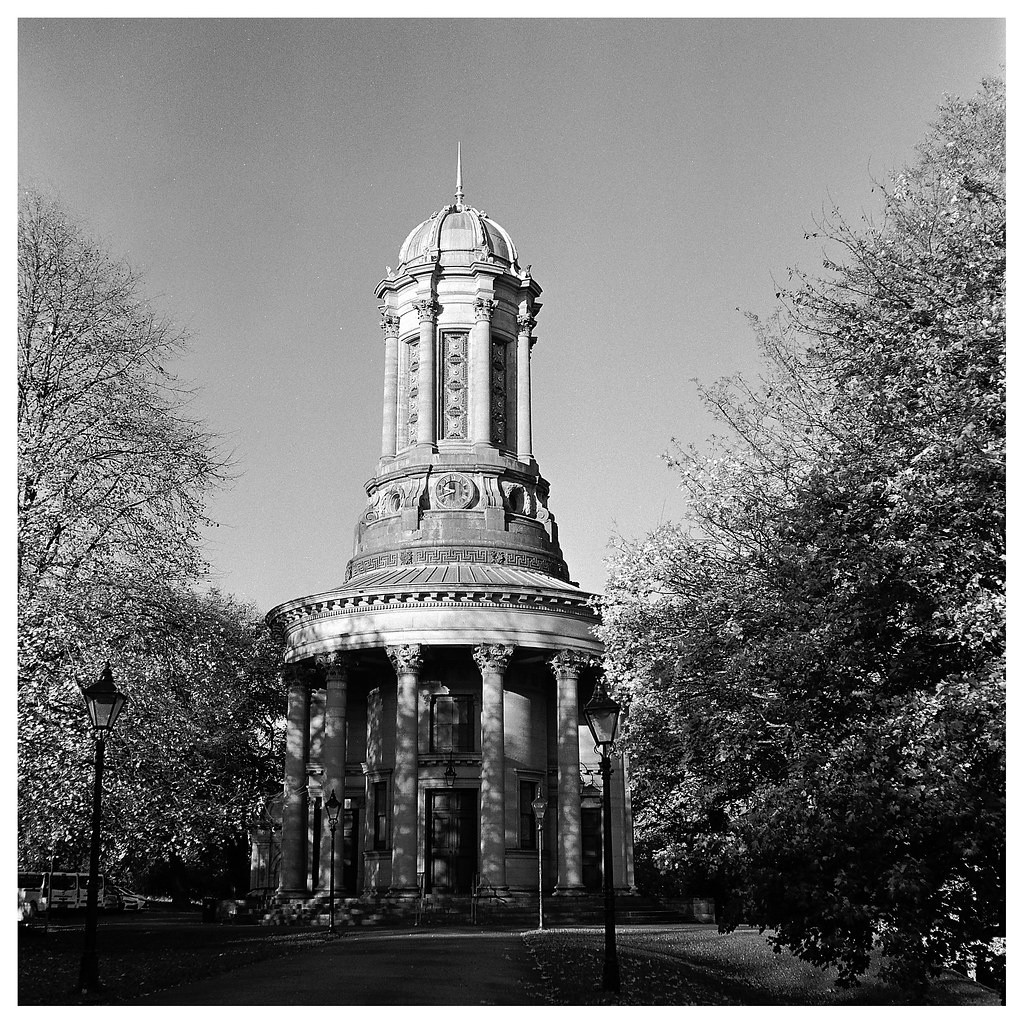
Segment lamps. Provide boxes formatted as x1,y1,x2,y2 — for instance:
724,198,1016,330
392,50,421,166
444,751,457,786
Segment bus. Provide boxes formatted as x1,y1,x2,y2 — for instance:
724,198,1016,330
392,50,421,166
18,871,126,914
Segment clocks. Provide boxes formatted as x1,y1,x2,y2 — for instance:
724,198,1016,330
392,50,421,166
435,472,475,509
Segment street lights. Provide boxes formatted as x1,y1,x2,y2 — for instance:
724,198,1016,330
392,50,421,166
77,660,129,990
583,673,621,996
324,788,342,936
531,786,549,931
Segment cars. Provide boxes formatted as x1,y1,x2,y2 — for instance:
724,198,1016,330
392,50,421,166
116,885,151,913
